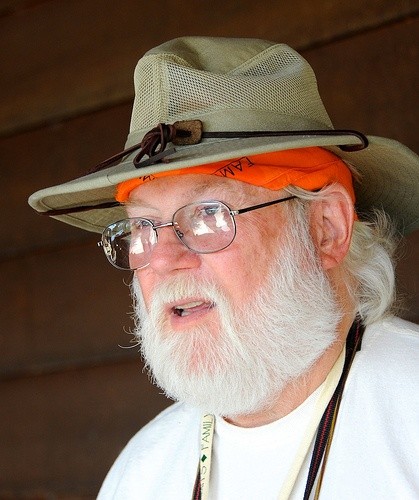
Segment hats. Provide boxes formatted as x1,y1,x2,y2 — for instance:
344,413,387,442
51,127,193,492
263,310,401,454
27,35,419,240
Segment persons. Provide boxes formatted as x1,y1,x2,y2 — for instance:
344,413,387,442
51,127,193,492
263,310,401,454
27,35,418,500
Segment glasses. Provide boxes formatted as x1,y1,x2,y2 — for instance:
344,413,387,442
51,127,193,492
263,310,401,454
96,188,296,270
